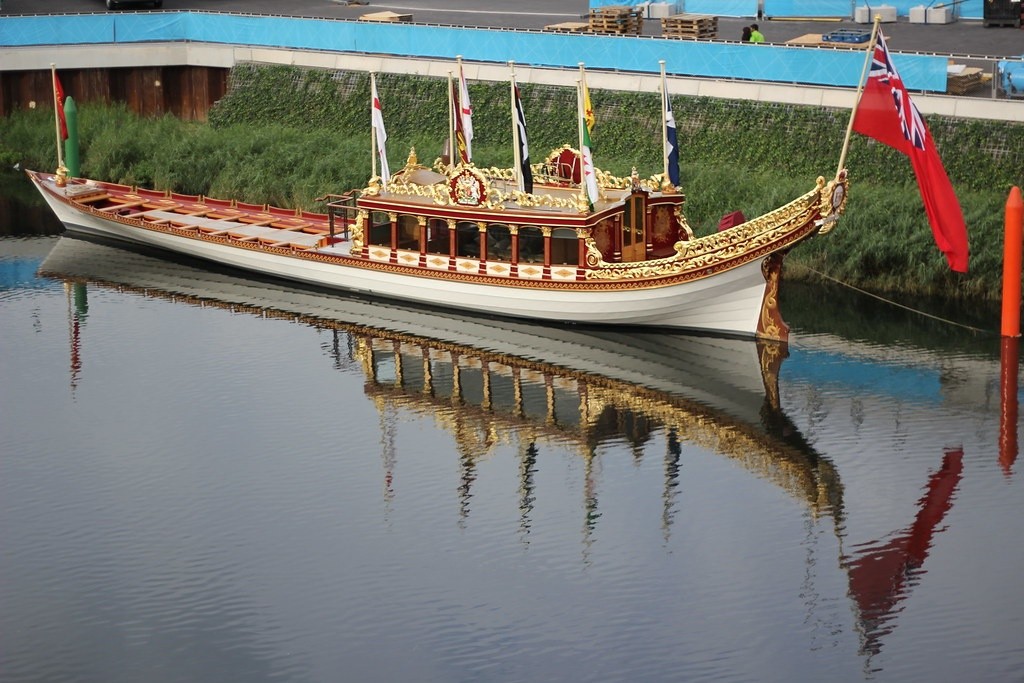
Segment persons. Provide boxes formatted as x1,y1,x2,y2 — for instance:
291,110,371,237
742,27,752,41
750,23,765,42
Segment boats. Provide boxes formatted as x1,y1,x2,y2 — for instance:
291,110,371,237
14,12,883,343
30,229,848,589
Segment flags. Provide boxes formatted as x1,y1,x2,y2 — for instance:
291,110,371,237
578,74,599,214
451,61,474,164
371,75,391,195
854,25,969,272
512,81,534,194
53,67,70,141
661,72,680,187
